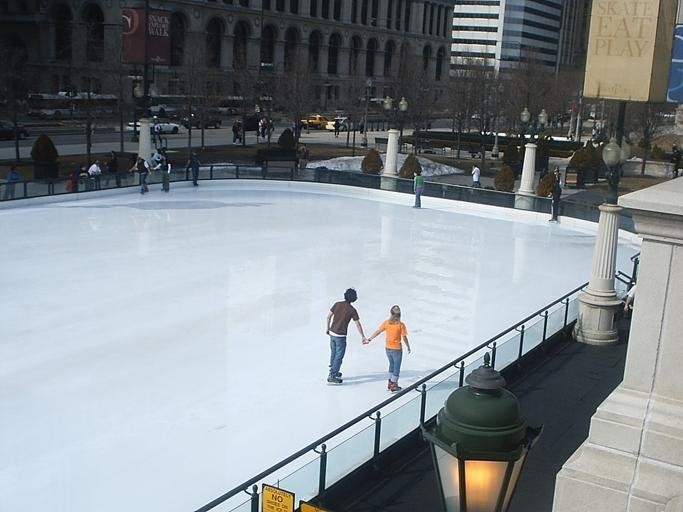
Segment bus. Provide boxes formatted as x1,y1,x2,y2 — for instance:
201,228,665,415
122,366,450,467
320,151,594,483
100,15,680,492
28,91,118,120
148,93,273,115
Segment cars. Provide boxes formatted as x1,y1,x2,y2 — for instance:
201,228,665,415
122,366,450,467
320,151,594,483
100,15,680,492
325,115,354,132
301,114,328,129
127,116,180,134
232,117,261,131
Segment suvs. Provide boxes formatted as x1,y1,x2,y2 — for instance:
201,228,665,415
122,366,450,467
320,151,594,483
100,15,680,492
183,111,222,129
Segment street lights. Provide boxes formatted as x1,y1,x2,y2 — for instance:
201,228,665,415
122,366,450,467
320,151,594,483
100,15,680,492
419,352,544,511
382,95,408,128
517,107,547,142
603,134,631,204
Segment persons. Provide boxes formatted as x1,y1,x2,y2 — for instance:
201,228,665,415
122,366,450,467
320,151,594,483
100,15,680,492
363,304,411,392
411,170,423,208
325,287,367,383
87,160,102,190
102,150,119,188
295,143,309,180
547,179,561,224
127,157,151,195
471,162,482,189
237,120,242,144
65,173,76,192
257,118,267,142
184,151,201,186
266,119,274,142
77,164,93,192
332,120,339,138
3,166,18,200
149,154,172,194
231,121,237,144
358,117,364,135
671,142,683,180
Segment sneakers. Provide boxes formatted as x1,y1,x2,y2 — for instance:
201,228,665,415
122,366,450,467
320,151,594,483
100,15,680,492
413,206,419,208
549,218,557,221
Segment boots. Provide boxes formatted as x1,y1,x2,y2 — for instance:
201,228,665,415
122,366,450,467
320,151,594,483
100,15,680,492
388,379,401,391
327,369,342,383
